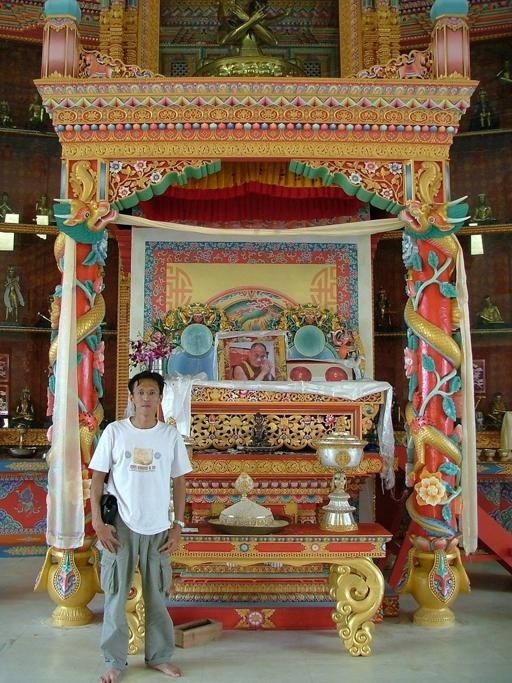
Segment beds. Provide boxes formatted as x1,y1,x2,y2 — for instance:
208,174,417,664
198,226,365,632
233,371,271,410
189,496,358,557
138,303,383,449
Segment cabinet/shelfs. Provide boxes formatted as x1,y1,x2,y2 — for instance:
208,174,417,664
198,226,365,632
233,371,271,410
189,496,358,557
374,126,512,431
0,126,62,450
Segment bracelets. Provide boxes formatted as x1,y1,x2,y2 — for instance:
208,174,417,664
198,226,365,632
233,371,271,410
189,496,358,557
173,519,185,528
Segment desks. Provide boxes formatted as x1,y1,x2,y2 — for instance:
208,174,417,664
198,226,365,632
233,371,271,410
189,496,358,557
112,521,394,657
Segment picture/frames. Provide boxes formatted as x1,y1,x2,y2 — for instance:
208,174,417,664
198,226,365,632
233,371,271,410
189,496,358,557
216,330,290,382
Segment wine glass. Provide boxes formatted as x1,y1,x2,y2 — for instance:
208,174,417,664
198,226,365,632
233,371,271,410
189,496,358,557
498,449,510,461
483,449,496,462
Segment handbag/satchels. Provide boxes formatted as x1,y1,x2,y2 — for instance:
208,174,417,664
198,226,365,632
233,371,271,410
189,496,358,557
100,494,118,525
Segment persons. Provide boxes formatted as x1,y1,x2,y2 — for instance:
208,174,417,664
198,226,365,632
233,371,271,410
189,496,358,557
231,343,275,381
8,385,39,428
0,89,46,129
468,89,508,429
87,371,194,683
3,263,27,320
0,190,53,226
